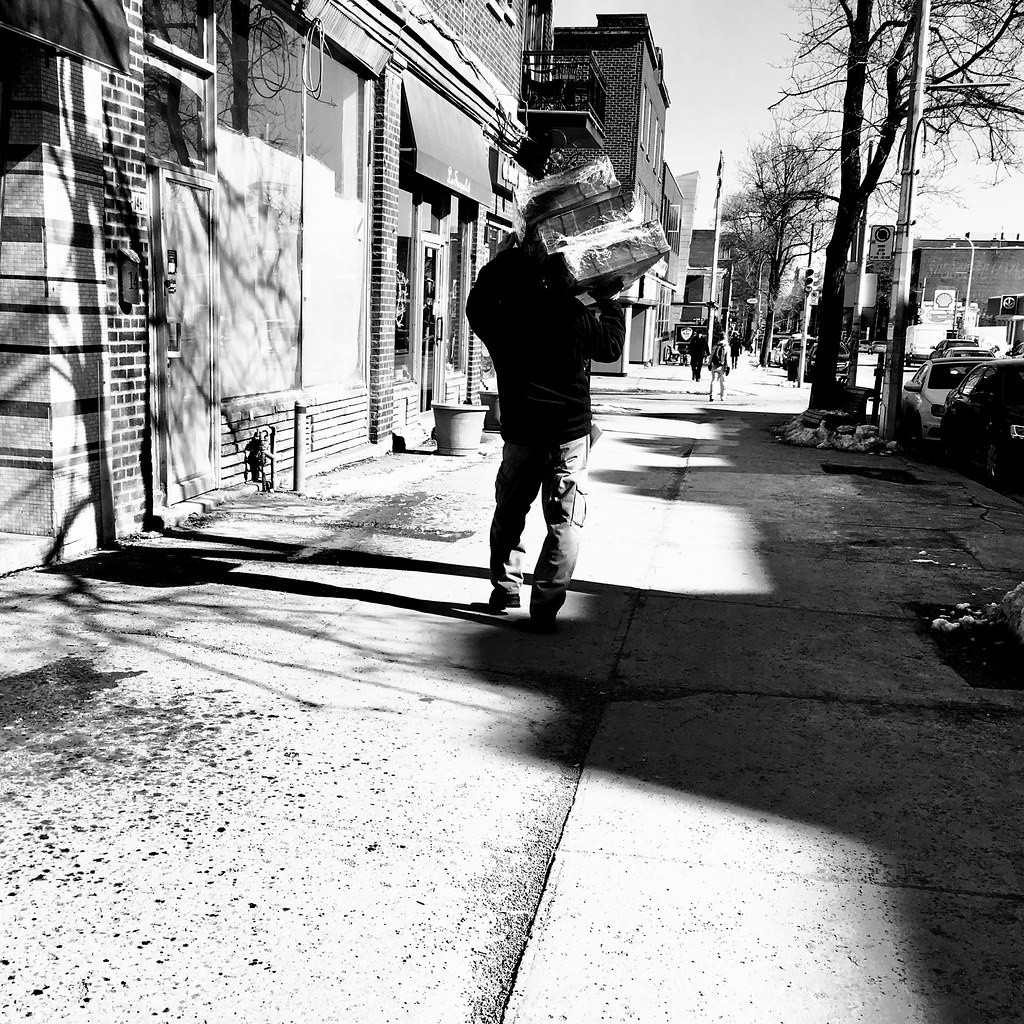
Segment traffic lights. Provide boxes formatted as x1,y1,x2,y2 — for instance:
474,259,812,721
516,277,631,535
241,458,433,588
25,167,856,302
805,269,814,294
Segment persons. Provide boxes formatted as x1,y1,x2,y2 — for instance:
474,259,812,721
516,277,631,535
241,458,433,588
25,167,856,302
687,330,743,402
466,232,626,636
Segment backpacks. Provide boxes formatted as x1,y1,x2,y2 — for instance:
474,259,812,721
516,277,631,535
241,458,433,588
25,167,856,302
713,343,726,365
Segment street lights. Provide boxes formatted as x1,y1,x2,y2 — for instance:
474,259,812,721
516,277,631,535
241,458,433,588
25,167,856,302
956,235,974,329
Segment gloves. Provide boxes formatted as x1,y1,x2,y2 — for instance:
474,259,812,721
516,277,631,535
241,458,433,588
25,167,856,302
726,367,730,376
708,363,712,371
588,276,624,301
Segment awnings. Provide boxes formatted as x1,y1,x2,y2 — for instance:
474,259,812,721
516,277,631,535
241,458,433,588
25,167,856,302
402,70,492,208
0,0,131,77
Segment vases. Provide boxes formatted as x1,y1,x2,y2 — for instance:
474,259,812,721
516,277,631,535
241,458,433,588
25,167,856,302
431,403,490,456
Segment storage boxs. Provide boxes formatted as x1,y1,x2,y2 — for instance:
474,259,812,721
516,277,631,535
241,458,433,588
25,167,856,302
478,391,501,431
537,192,644,256
513,153,622,244
562,218,672,306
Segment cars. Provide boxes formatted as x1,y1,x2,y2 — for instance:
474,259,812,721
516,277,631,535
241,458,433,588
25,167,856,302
899,325,1024,492
858,339,887,354
752,324,851,380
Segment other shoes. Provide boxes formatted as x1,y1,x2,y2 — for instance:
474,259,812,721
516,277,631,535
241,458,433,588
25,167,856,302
489,589,521,609
532,609,556,634
